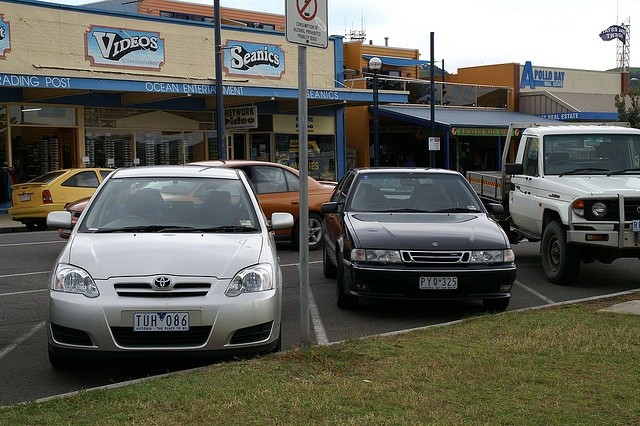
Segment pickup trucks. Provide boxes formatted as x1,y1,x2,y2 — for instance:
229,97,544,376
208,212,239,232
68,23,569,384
465,120,640,284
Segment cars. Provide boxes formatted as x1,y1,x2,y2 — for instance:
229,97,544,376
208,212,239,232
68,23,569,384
320,166,518,313
60,158,336,251
46,165,296,368
8,167,115,229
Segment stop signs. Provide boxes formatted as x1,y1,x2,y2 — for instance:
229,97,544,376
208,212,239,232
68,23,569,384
286,0,329,48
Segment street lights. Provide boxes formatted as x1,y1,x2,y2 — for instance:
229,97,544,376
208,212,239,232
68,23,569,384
367,56,384,166
